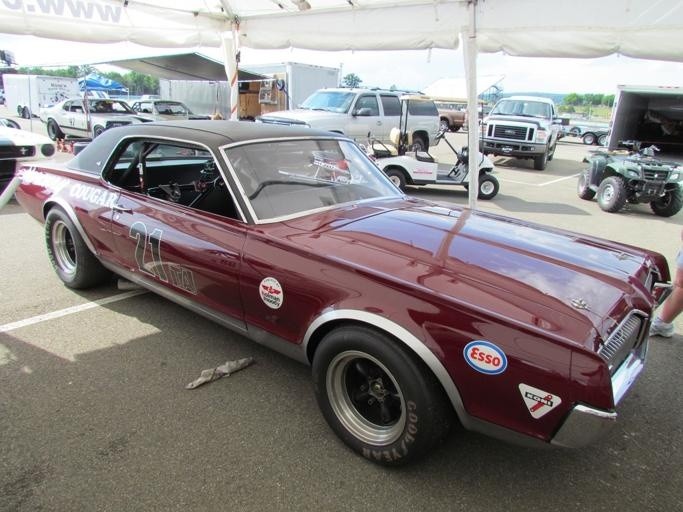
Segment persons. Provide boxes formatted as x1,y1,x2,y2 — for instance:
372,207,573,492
649,228,683,338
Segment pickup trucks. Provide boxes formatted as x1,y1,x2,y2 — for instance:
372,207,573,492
254,86,439,154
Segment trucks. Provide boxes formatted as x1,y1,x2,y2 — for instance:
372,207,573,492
2,73,108,118
603,84,682,186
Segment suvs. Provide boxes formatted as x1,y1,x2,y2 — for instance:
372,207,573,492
402,95,611,145
575,139,683,217
477,95,558,171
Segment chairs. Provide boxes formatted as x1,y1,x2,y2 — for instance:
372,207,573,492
189,176,237,218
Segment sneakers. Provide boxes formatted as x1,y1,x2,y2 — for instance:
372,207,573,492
648,315,674,338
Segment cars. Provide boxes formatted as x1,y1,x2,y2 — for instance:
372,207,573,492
0,107,55,188
15,119,672,465
126,99,211,120
40,97,153,140
0,89,4,103
